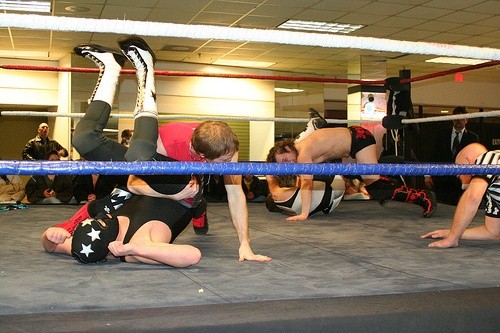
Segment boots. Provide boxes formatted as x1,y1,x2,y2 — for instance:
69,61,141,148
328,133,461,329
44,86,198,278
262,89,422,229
364,176,437,218
293,108,328,144
116,36,158,120
74,44,128,110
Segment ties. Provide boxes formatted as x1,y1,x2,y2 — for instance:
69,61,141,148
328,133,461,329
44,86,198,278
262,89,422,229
452,131,461,162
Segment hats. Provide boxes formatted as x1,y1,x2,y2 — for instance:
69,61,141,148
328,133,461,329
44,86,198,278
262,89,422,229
121,130,132,137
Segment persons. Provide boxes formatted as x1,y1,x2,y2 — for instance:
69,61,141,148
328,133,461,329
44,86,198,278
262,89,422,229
23,34,273,269
262,105,442,222
423,106,479,206
419,141,498,249
